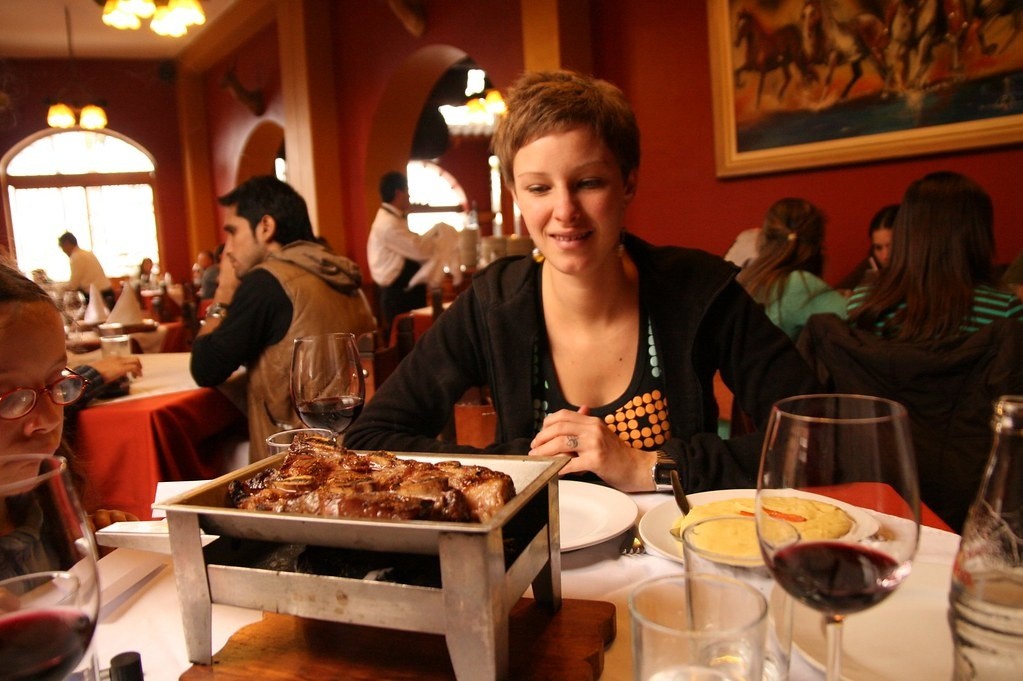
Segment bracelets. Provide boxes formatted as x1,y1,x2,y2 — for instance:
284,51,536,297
207,302,229,318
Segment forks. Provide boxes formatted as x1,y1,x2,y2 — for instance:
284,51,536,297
619,529,644,555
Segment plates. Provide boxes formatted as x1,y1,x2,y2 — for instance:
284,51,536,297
638,489,878,576
558,479,637,551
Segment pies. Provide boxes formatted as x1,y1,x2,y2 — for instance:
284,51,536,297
682,496,852,558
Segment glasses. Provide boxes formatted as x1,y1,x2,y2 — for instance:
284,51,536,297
0,366,91,421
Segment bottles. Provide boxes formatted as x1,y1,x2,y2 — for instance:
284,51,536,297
945,397,1023,680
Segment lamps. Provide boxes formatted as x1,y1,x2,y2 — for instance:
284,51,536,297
42,4,109,129
102,0,205,37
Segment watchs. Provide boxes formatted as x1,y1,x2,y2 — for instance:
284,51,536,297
652,450,677,492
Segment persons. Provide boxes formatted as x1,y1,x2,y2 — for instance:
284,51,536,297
855,205,901,276
340,71,820,494
712,197,846,441
191,177,371,476
0,264,139,610
193,243,227,296
62,354,142,421
58,231,115,310
33,269,52,283
848,172,1023,341
136,258,153,281
367,172,437,329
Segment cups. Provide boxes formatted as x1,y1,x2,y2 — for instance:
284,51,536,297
0,453,101,681
100,335,132,380
627,573,767,681
681,517,802,680
265,427,333,456
290,332,365,438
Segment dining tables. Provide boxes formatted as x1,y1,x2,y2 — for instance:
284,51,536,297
0,286,961,681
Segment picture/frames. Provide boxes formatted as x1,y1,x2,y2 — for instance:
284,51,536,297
707,0,1023,179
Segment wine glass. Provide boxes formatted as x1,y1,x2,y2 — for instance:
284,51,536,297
61,292,87,340
754,394,921,680
